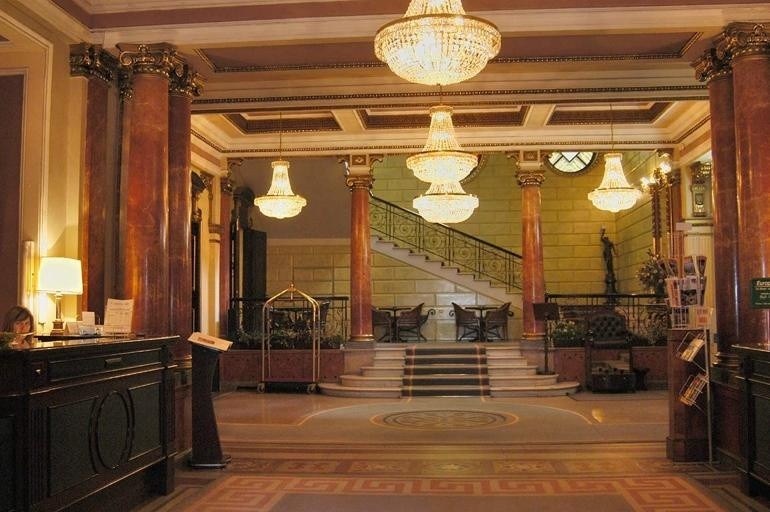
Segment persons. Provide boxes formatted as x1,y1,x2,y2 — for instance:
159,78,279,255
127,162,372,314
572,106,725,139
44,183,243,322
599,226,619,275
1,306,35,350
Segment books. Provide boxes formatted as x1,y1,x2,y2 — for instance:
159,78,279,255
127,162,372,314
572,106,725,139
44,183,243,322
679,373,708,406
680,337,704,362
657,254,714,329
187,331,236,353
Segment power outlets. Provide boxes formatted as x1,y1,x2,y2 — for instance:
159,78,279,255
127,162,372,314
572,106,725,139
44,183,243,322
371,0,504,88
411,179,482,226
407,85,479,184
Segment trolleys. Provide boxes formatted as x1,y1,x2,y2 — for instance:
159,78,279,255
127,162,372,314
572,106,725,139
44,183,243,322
256,282,325,395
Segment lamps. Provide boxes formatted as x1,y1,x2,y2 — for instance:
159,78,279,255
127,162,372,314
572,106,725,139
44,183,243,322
34,255,88,336
248,113,309,220
583,101,643,215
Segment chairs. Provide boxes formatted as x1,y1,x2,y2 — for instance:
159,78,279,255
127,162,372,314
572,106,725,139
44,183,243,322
451,301,512,342
254,301,331,338
369,300,430,343
581,308,637,393
